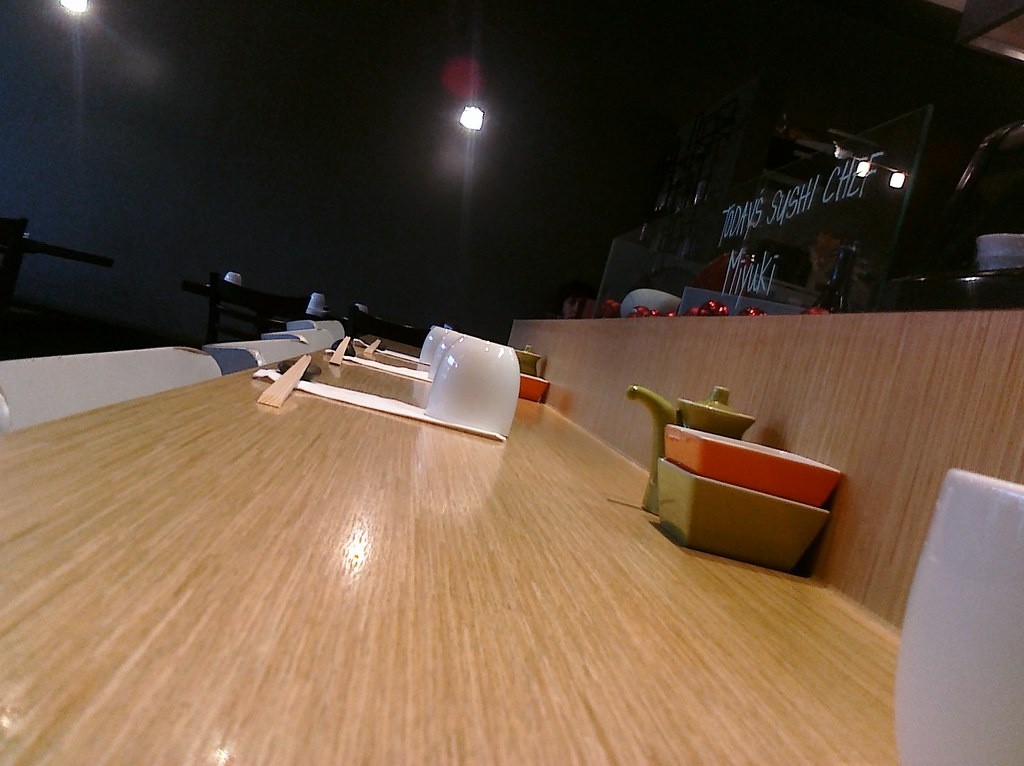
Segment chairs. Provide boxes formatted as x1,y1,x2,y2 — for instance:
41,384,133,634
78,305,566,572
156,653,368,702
205,272,461,359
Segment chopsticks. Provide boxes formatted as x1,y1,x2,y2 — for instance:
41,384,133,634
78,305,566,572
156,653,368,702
258,355,312,408
330,336,351,366
365,339,381,354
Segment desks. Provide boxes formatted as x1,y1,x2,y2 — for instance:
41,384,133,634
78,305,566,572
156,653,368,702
0,238,114,314
181,280,289,321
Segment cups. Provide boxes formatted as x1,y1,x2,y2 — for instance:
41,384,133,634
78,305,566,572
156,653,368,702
224,271,245,289
890,467,1024,766
974,233,1023,272
420,325,446,365
424,336,521,438
428,331,462,380
305,292,325,318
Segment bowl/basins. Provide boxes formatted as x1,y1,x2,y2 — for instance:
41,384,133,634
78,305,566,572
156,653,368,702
657,458,829,573
664,425,840,508
519,373,549,401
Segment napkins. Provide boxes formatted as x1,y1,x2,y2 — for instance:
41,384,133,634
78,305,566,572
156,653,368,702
355,339,431,366
253,369,506,442
325,349,433,383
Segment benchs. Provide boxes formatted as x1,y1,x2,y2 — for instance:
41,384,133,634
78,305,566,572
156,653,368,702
0,334,903,766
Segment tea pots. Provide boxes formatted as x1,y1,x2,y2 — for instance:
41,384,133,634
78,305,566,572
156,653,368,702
516,346,542,377
629,381,755,515
